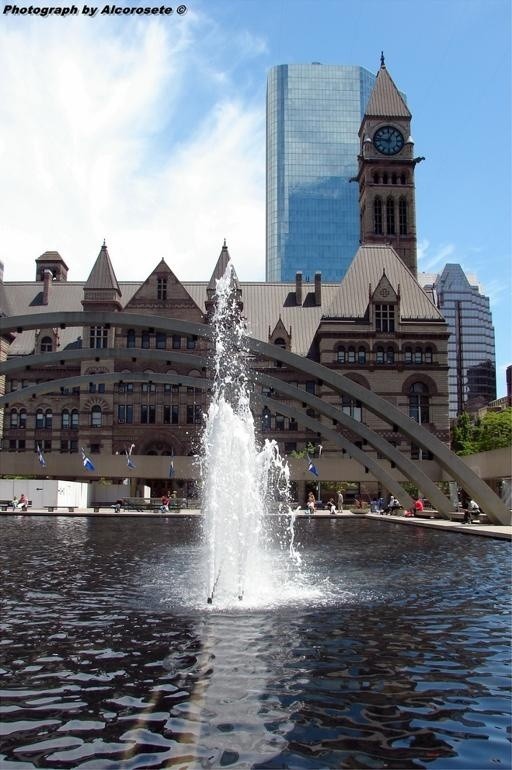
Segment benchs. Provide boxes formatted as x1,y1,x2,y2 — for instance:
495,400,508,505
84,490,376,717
0,499,491,526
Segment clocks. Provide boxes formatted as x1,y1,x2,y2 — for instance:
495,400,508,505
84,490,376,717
372,125,404,156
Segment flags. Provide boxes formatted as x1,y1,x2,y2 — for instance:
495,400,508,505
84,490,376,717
170,461,175,477
81,451,95,472
37,445,47,466
128,459,136,469
307,456,318,476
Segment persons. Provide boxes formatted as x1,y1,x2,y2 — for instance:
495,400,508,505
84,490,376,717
115,499,122,513
160,496,169,513
410,498,423,516
337,491,343,513
462,497,480,524
18,494,28,509
307,497,315,513
308,492,315,512
12,496,18,510
381,496,400,515
